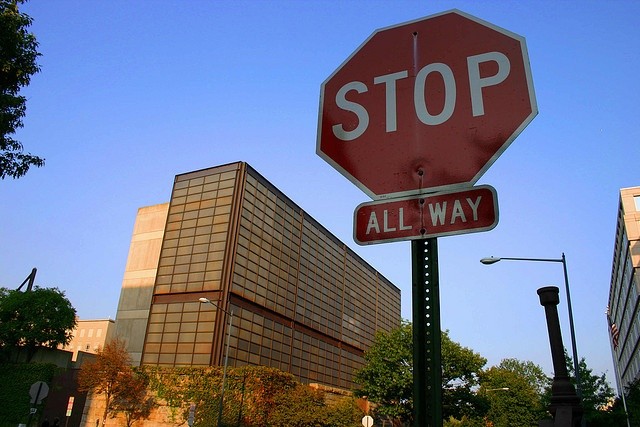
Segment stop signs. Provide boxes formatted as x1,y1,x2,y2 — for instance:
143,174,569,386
316,7,539,201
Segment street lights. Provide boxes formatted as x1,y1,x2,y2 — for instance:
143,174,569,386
198,296,235,427
480,252,582,399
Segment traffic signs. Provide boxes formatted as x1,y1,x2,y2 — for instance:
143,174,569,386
352,184,498,246
